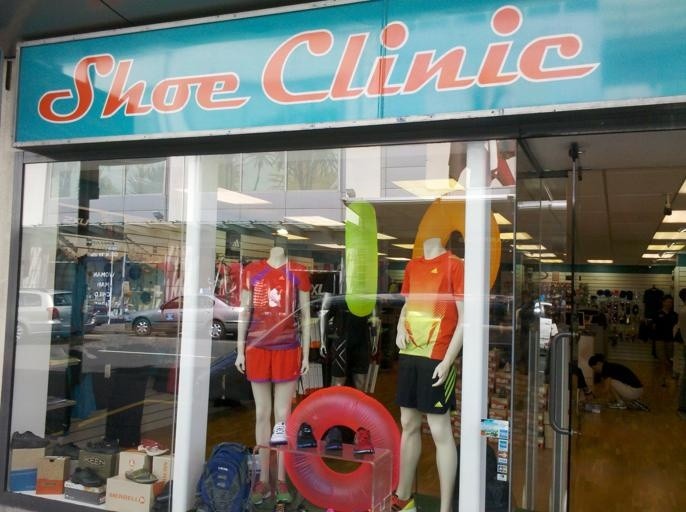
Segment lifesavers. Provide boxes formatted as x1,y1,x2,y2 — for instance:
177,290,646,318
282,386,400,511
413,191,501,289
346,200,378,317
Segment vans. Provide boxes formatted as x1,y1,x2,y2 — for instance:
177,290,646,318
210,293,511,432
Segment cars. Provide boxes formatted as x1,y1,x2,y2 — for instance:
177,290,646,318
15,290,95,343
124,295,244,341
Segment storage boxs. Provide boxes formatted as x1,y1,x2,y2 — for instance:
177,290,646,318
421,349,577,451
8,448,172,511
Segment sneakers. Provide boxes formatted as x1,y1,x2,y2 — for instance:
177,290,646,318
86,440,119,454
71,467,104,486
249,481,270,504
606,398,628,410
274,481,293,503
11,431,50,448
295,422,318,448
323,428,343,453
271,422,288,444
626,399,651,412
137,438,171,457
53,442,81,458
352,426,376,455
366,490,416,512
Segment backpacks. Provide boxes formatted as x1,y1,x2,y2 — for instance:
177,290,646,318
195,441,256,512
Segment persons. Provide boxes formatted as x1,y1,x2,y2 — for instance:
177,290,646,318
587,352,651,413
318,270,380,393
231,244,313,506
576,368,594,402
677,288,686,414
390,236,466,512
650,294,679,387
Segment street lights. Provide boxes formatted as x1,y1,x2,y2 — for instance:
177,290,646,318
105,251,118,327
277,229,290,315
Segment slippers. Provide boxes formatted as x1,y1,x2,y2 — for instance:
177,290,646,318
125,468,159,483
